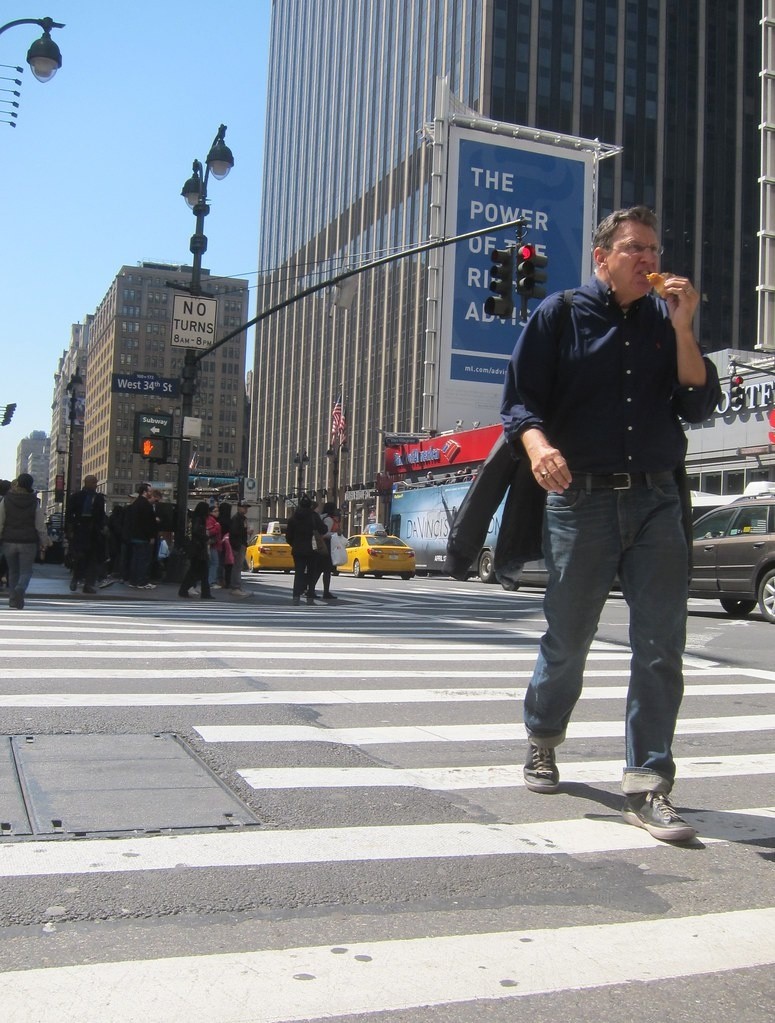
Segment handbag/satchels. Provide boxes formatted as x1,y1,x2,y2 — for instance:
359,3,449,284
66,520,93,572
158,539,169,558
312,512,328,554
224,539,234,564
331,533,349,566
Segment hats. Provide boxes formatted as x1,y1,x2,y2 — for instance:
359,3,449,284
238,499,252,507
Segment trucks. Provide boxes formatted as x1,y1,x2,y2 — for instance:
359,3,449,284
688,480,775,524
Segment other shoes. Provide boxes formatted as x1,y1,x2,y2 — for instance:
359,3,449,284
323,594,337,599
306,602,316,605
15,586,25,609
313,595,320,599
230,588,247,595
69,572,156,594
8,601,16,607
178,593,192,598
210,584,222,589
202,596,215,599
188,587,198,595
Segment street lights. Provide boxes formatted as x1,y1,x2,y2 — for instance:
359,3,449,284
294,450,310,509
324,436,350,515
166,122,235,577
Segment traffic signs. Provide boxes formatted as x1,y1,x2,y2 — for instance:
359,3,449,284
133,412,174,457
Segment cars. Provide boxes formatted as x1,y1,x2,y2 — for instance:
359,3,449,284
242,521,296,574
500,559,622,592
336,523,416,581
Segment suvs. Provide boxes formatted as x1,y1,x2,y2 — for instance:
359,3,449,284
687,491,775,625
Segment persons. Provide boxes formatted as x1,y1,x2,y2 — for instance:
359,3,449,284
285,498,344,605
500,206,721,840
64,475,254,595
0,474,48,610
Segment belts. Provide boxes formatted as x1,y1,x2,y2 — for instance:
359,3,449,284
565,471,674,490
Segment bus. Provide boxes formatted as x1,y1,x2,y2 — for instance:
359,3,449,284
387,473,511,584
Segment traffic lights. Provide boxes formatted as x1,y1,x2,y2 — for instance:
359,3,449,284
730,375,744,408
528,253,548,299
140,436,168,461
484,247,516,319
517,244,536,297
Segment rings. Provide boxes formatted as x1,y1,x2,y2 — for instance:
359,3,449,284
542,472,549,478
686,287,692,293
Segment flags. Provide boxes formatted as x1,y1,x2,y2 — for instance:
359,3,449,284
331,396,346,444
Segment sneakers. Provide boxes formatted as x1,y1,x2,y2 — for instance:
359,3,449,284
621,790,695,840
523,745,560,794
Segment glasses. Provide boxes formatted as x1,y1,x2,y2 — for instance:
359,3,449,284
607,241,665,257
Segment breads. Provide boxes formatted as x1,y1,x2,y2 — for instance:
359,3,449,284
644,273,677,299
